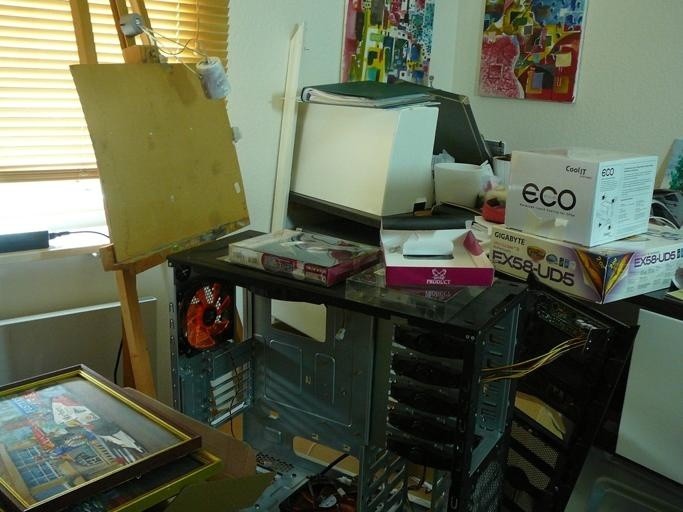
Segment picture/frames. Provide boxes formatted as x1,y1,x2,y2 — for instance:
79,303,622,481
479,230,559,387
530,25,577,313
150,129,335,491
478,0,588,103
0,363,197,512
340,0,434,87
45,448,223,511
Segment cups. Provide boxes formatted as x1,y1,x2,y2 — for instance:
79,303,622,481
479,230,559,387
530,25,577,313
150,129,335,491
434,162,488,205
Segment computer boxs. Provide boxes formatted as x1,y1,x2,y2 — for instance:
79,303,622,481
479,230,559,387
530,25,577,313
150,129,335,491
164,227,639,512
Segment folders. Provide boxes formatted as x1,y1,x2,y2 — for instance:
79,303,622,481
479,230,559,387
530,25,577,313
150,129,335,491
435,88,492,167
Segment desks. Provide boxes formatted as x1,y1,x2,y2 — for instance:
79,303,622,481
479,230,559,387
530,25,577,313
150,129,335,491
508,268,639,512
169,228,524,512
531,285,683,493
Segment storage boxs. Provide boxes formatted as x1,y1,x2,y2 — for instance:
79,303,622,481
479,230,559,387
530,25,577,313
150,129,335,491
505,145,657,247
491,224,683,301
